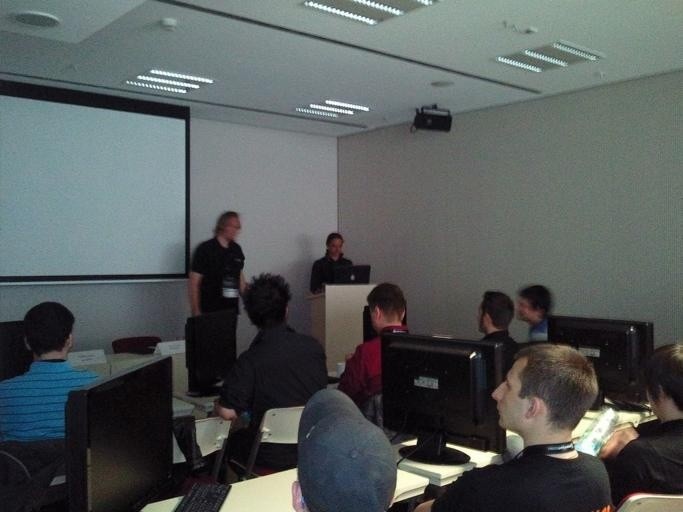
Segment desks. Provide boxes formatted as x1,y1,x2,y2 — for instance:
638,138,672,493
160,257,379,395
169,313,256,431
0,320,683,512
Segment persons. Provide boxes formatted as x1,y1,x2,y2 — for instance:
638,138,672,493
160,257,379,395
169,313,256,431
338,283,408,407
214,273,326,469
598,344,682,508
0,302,99,441
311,233,353,295
517,285,551,344
477,291,517,346
189,212,250,317
414,344,611,511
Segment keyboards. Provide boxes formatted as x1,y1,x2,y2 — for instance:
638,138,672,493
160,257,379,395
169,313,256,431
174,481,232,512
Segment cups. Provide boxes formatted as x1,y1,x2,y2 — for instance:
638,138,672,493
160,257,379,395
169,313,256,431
336,362,346,379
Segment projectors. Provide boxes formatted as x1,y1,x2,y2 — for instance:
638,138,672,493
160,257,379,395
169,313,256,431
414,113,452,131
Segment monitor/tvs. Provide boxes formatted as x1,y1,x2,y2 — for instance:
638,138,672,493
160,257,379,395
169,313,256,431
380,332,507,465
186,308,237,397
547,313,653,411
363,301,407,346
64,355,173,512
0,321,34,381
334,264,371,283
